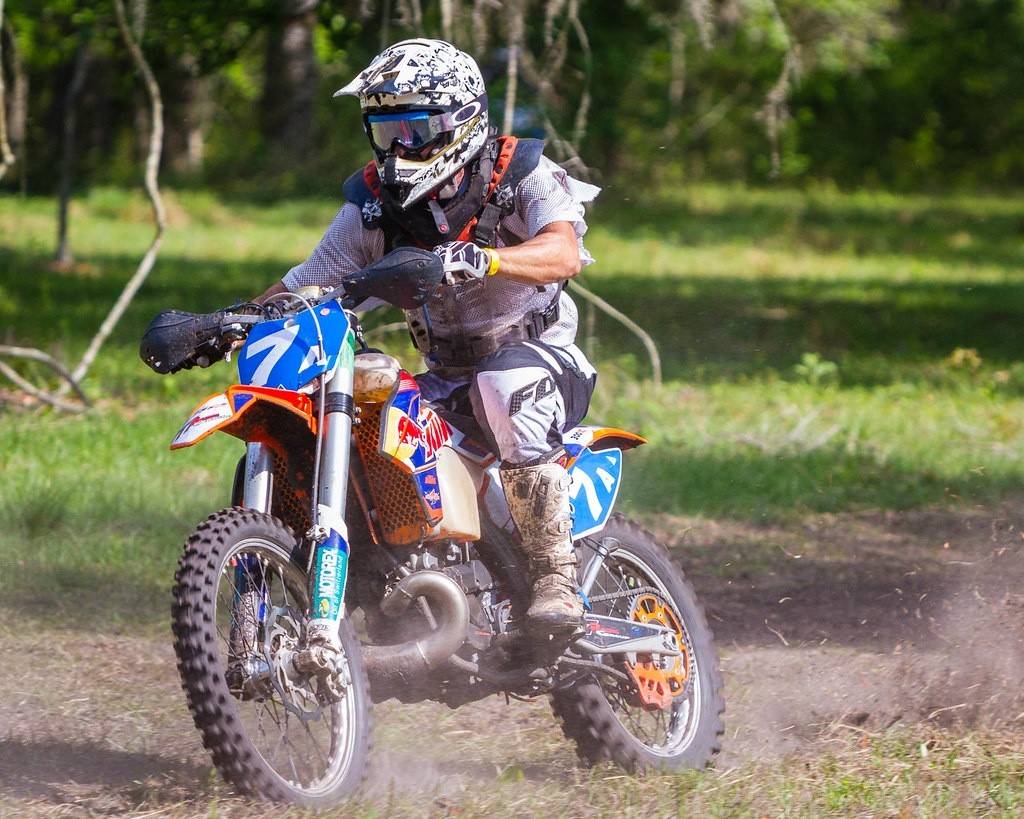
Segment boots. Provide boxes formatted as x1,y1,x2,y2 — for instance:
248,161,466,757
498,445,586,636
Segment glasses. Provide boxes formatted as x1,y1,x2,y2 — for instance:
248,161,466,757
368,112,456,154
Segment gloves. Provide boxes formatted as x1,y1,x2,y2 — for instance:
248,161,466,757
432,241,489,286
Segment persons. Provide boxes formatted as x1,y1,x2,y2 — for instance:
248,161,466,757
196,36,599,630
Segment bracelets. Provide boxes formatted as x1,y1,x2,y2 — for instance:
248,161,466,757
484,247,500,276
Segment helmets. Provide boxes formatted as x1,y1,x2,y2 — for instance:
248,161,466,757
333,38,488,213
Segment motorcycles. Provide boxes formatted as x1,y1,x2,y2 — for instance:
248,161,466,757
139,245,726,812
